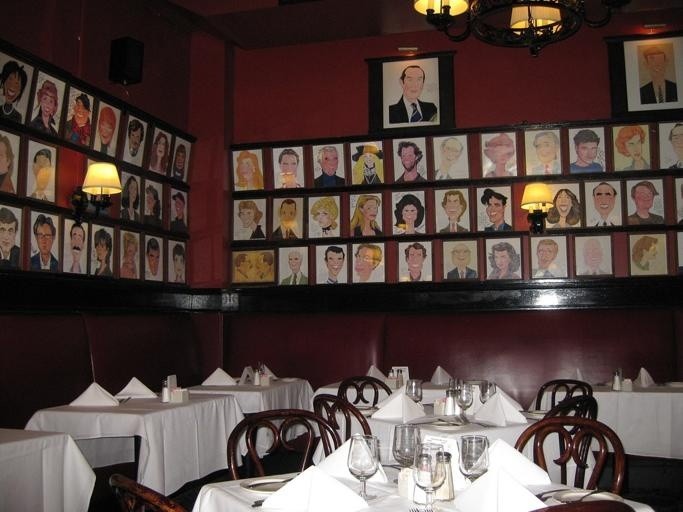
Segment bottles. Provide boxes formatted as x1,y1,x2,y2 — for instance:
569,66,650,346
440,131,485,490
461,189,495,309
253,369,260,387
398,370,403,388
389,369,395,379
161,381,168,403
612,371,621,391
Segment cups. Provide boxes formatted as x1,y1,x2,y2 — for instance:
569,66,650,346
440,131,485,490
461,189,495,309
346,379,496,512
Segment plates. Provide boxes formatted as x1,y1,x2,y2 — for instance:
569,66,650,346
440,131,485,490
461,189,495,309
279,378,300,382
665,382,683,388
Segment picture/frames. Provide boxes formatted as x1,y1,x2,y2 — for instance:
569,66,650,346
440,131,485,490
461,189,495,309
604,29,683,118
364,49,456,131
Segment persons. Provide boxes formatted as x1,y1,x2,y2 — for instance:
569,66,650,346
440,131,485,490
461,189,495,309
640,47,676,104
388,64,437,124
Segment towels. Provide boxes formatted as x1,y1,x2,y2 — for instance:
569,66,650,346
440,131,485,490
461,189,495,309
200,367,237,386
632,366,655,389
430,366,454,385
366,365,387,385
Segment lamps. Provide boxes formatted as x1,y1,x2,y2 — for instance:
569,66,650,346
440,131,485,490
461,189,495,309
509,0,562,59
413,0,474,43
